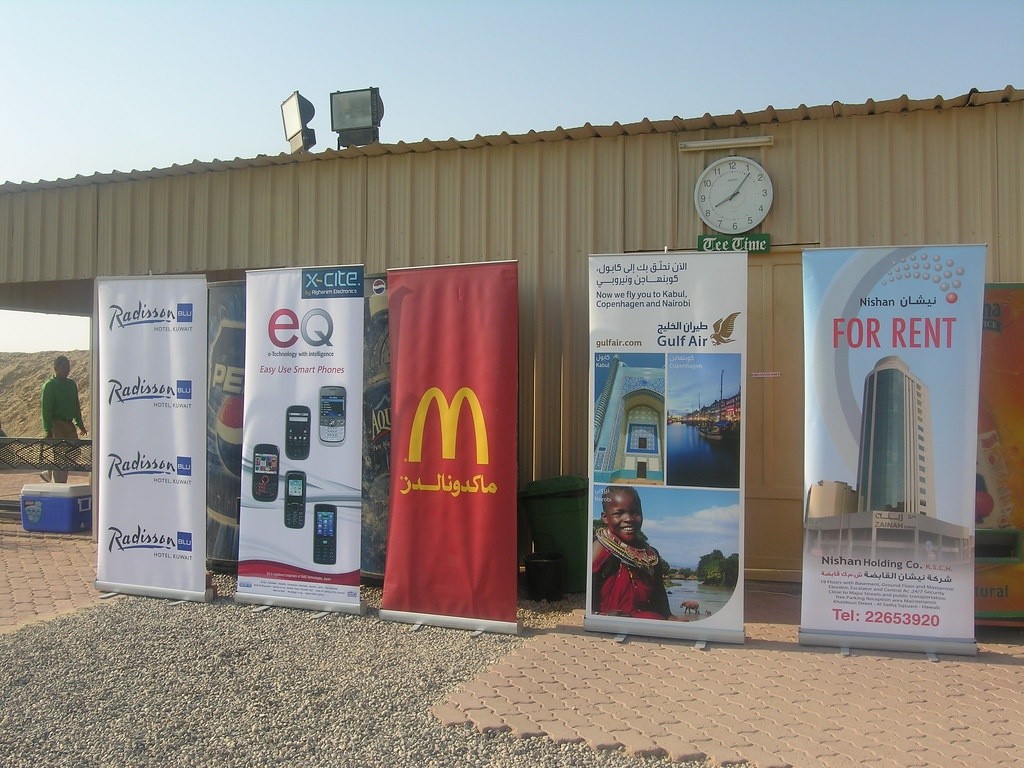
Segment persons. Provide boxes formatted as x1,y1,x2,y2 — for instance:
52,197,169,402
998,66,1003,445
592,485,689,622
40,355,88,484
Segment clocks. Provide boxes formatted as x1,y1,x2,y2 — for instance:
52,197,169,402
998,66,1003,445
693,157,775,235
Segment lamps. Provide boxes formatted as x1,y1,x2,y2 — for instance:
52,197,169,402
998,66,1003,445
281,91,316,153
330,87,385,149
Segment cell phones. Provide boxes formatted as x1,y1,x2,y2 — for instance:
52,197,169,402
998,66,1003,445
284,471,306,529
252,444,280,502
285,405,311,460
313,504,338,564
319,386,346,447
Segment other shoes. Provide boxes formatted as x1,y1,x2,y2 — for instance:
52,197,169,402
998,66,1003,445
40,470,53,483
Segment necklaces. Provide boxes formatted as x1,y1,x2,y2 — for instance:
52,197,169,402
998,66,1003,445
595,528,658,568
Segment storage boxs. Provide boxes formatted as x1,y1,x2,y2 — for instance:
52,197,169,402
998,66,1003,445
20,483,93,533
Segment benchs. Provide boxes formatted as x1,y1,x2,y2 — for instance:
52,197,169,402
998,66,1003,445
0,437,92,475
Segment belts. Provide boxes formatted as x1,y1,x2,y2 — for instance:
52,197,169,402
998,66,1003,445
59,419,74,422
